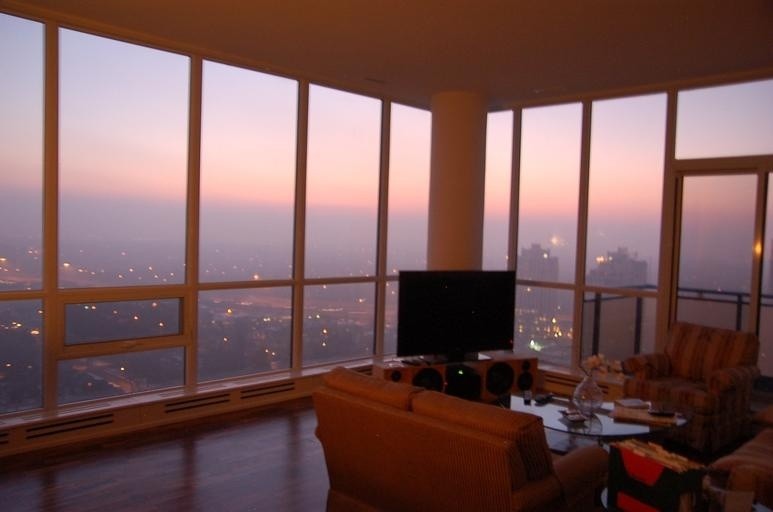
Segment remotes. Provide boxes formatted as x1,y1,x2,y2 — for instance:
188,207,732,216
647,408,675,417
535,390,556,403
524,389,533,404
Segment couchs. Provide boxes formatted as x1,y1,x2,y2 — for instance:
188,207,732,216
308,364,611,511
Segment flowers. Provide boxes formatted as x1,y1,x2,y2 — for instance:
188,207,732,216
575,352,608,409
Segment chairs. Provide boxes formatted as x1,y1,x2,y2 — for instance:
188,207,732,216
620,320,762,451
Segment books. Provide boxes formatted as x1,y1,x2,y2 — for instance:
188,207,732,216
614,397,650,409
614,405,677,428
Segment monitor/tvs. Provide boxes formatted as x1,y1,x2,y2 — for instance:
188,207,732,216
396,271,518,364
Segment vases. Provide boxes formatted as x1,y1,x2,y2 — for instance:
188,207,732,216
573,374,603,410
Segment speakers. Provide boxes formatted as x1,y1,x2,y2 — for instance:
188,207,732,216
373,355,537,403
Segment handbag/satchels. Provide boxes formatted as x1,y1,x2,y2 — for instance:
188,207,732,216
607,436,706,511
